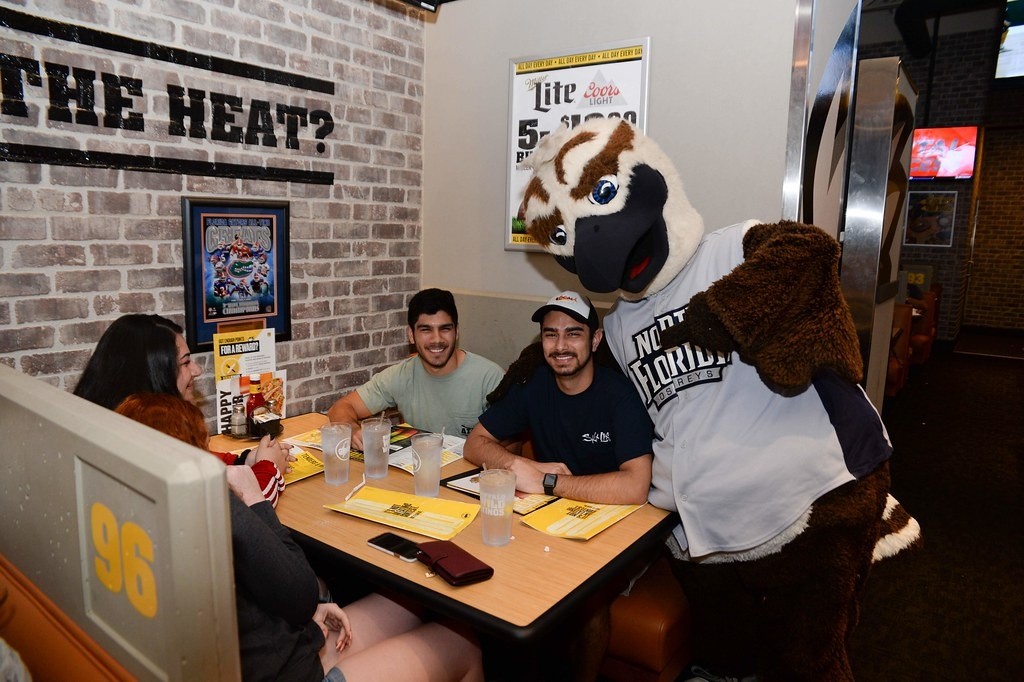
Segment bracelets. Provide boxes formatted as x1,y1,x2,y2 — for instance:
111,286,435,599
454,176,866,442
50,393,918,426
239,449,251,465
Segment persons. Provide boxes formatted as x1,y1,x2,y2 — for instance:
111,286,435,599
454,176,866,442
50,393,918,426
71,313,298,509
328,287,504,451
906,283,928,336
116,390,484,682
463,291,655,682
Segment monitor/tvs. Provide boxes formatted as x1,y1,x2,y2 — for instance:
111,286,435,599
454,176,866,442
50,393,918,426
909,126,981,185
994,0,1024,79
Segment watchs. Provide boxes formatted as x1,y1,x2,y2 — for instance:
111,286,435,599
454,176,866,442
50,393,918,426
543,473,557,495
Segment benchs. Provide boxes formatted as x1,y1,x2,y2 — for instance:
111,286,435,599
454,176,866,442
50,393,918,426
1,365,244,682
888,306,913,396
408,290,696,682
909,292,938,364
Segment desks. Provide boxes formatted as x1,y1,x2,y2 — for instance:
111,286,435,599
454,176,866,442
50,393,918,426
208,413,676,682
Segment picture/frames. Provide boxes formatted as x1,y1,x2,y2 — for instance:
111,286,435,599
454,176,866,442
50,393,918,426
903,191,958,247
182,195,292,353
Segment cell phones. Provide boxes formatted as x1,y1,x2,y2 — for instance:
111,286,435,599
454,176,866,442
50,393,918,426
367,533,421,562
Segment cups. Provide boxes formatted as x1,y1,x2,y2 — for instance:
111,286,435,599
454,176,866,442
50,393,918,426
412,433,443,499
480,468,516,546
322,421,352,484
361,418,393,479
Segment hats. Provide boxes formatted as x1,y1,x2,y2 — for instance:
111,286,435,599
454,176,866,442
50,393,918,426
531,289,599,332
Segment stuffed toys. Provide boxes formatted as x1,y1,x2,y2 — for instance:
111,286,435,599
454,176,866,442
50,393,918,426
517,117,921,682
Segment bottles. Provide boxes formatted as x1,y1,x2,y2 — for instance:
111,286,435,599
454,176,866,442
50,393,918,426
247,373,265,433
231,404,246,435
268,399,279,415
